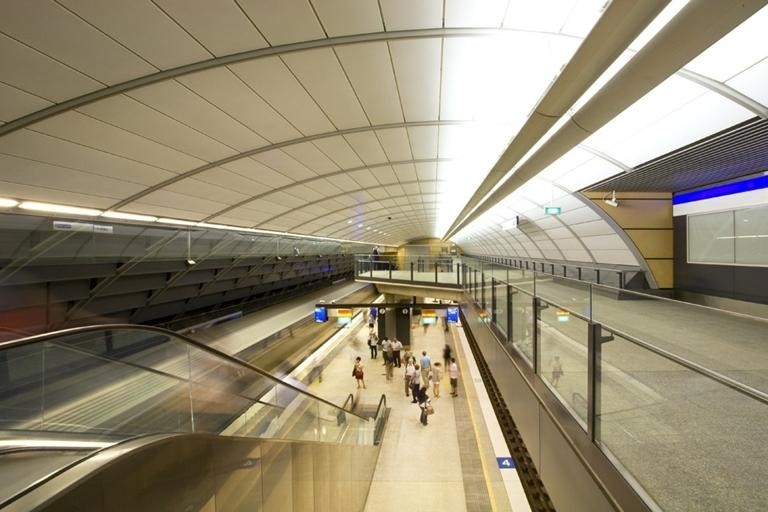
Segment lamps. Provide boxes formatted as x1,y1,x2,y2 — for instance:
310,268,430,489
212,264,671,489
185,229,196,267
318,242,322,258
342,243,345,257
275,238,282,261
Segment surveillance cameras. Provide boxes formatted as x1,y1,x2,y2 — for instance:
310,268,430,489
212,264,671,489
604,199,619,208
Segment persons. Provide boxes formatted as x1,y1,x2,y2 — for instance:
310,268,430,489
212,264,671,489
368,308,458,426
354,356,366,389
551,356,564,388
371,245,380,270
